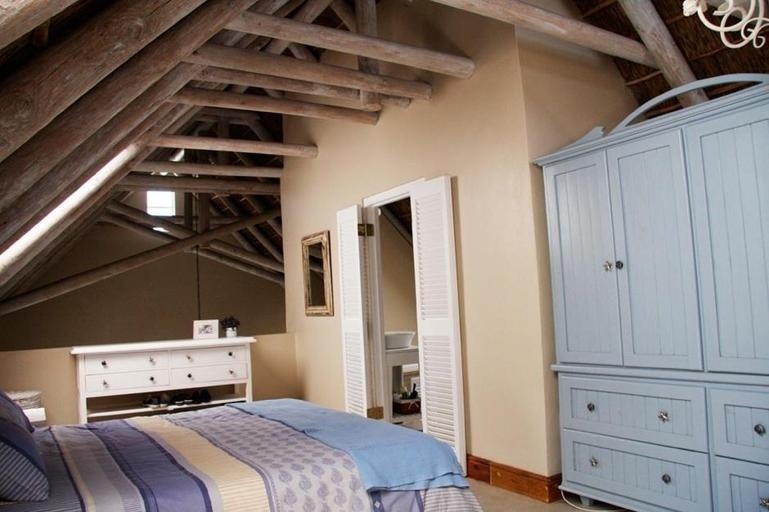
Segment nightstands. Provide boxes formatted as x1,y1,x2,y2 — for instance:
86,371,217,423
3,407,47,429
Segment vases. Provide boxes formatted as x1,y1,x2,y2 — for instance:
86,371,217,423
225,326,237,337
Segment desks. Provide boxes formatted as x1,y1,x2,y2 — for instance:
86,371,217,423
0,390,44,407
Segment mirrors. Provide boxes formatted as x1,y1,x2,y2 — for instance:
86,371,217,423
300,230,336,318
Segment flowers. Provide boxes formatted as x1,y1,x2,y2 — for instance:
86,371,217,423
219,315,241,331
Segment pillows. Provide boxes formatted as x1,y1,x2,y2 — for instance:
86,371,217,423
1,415,50,503
0,393,34,432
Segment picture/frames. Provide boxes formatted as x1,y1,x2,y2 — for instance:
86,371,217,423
192,319,219,338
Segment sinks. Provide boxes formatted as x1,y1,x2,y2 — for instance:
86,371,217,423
385,330,416,349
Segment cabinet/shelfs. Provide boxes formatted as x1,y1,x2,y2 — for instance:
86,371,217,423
703,382,769,511
540,83,713,372
551,366,714,512
69,335,258,424
684,75,769,376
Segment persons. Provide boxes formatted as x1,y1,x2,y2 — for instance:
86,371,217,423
199,324,213,334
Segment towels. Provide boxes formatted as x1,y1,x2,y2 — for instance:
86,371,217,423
224,397,470,494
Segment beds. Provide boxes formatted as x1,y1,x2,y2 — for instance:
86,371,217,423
0,390,483,512
